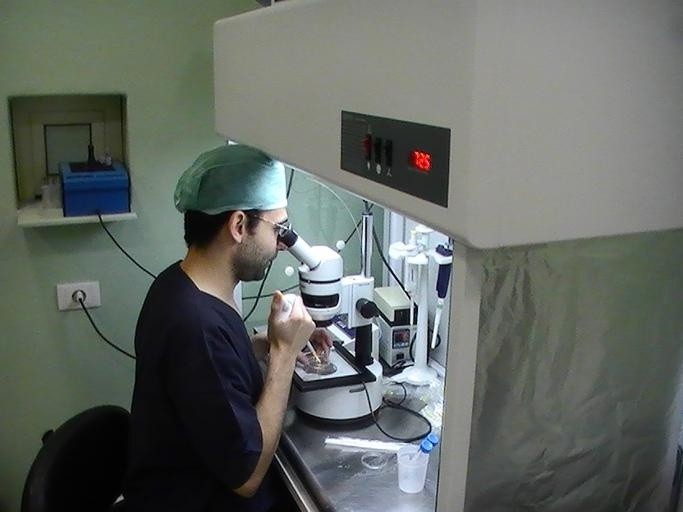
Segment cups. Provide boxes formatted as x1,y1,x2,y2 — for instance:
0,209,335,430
397,444,430,494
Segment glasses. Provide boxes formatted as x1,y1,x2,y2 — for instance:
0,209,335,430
252,215,293,237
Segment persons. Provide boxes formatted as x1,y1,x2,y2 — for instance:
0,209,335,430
121,142,333,511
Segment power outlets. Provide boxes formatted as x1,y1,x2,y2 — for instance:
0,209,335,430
56,281,103,314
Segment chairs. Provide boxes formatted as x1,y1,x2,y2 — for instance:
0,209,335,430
20,404,129,512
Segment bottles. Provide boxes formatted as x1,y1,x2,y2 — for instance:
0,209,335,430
411,432,439,461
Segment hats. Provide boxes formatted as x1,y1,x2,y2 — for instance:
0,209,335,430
175,144,287,216
